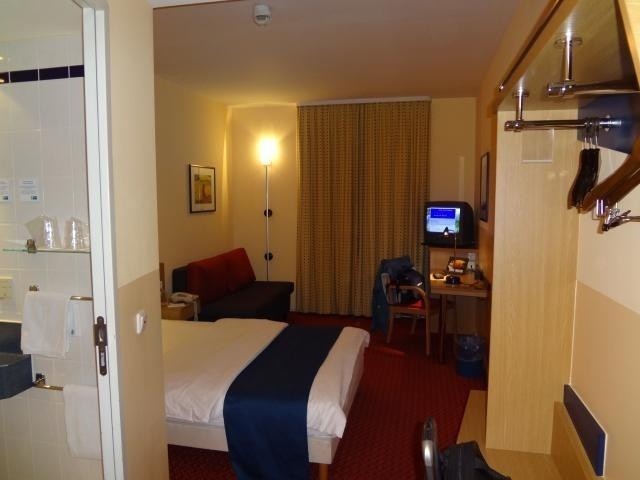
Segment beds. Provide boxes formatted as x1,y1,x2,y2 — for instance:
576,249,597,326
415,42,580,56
159,262,370,480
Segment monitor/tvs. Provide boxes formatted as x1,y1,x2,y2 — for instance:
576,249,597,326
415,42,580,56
423,200,475,246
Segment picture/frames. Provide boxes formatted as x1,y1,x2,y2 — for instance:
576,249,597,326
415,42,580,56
189,164,216,213
479,152,489,223
446,256,469,274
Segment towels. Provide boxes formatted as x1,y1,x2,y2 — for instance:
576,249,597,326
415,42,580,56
21,291,70,359
63,384,102,460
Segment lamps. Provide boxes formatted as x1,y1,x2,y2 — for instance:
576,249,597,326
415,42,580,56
444,226,457,274
256,135,279,281
253,5,271,26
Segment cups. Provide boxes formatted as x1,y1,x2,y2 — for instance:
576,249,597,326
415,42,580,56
463,272,472,285
40,218,85,250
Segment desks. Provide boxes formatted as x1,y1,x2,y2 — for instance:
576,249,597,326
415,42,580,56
431,280,488,364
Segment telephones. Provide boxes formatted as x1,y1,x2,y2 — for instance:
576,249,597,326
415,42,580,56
170,292,200,303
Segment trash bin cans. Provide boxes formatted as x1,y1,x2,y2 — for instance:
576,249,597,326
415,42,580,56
453,335,486,377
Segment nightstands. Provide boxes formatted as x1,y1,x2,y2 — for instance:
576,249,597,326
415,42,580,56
161,294,200,320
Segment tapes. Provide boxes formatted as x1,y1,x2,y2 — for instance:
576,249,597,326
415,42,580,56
446,275,460,284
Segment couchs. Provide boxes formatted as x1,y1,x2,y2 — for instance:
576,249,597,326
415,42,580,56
173,247,294,322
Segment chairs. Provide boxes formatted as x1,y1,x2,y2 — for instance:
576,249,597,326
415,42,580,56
382,273,455,356
